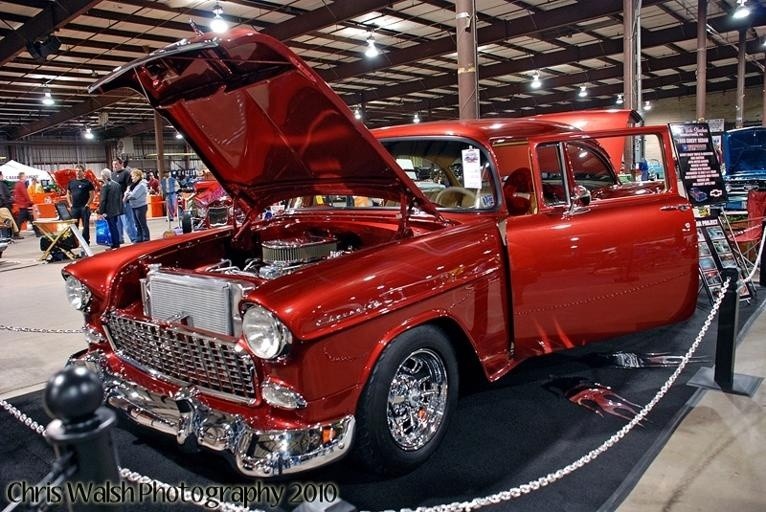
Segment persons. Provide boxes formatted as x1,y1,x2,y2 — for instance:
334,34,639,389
1,157,206,251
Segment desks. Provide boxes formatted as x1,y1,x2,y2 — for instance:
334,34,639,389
33,217,78,262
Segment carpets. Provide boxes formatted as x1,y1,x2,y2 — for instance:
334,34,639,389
0,276,766,512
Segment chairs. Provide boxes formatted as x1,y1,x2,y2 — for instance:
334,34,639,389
495,165,566,219
720,189,766,273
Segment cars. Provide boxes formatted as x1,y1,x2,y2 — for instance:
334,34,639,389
60,17,699,481
703,125,766,218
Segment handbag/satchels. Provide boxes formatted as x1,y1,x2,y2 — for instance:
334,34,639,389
95,220,113,246
